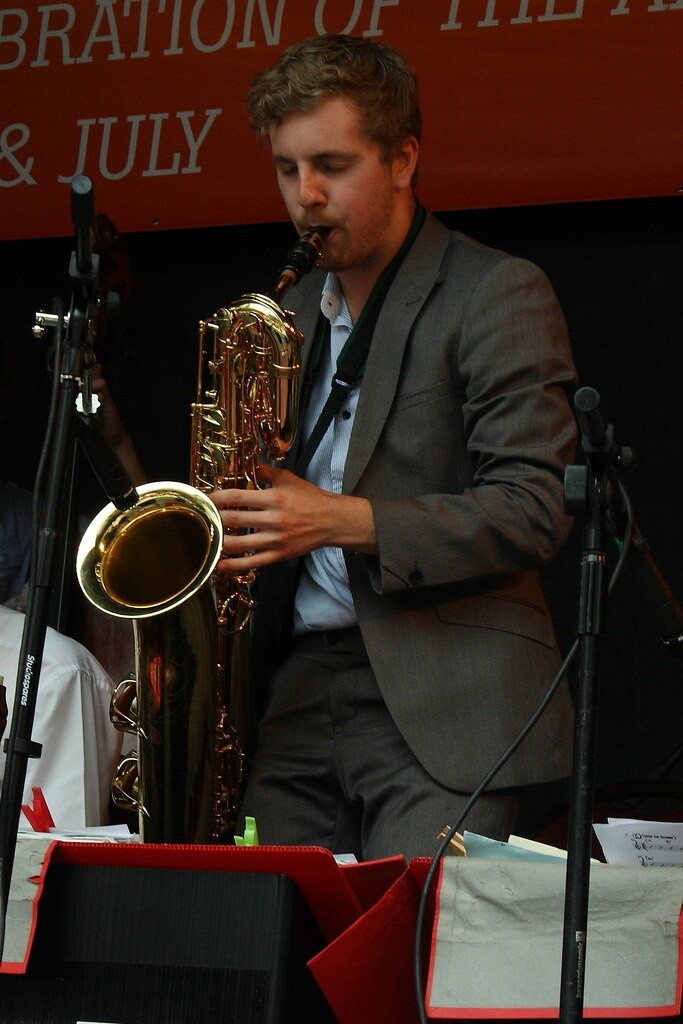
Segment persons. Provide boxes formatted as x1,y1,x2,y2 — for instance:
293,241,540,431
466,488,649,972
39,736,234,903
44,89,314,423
1,484,125,834
206,36,575,858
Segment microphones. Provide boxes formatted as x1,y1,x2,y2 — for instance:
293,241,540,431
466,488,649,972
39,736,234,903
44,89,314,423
606,485,683,666
77,414,140,512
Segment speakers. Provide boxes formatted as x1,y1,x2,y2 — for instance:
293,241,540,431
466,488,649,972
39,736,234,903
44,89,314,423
0,861,332,1024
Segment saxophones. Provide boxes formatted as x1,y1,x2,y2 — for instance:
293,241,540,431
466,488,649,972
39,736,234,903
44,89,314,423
74,226,335,845
